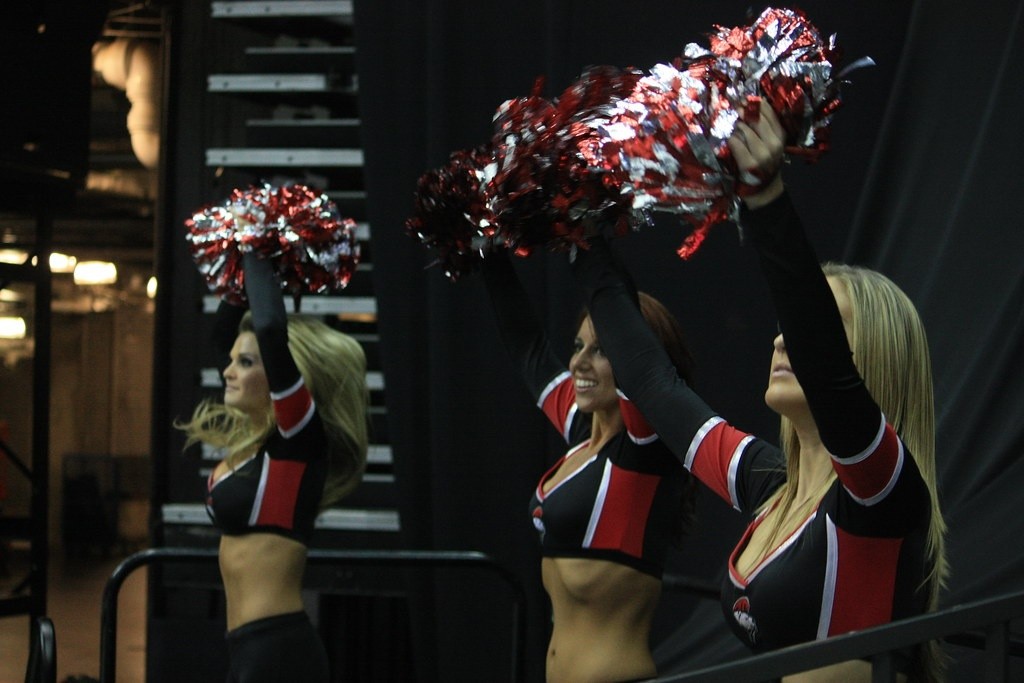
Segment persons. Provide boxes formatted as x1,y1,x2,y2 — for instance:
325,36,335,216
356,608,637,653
475,212,701,683
583,95,945,683
176,240,369,683
